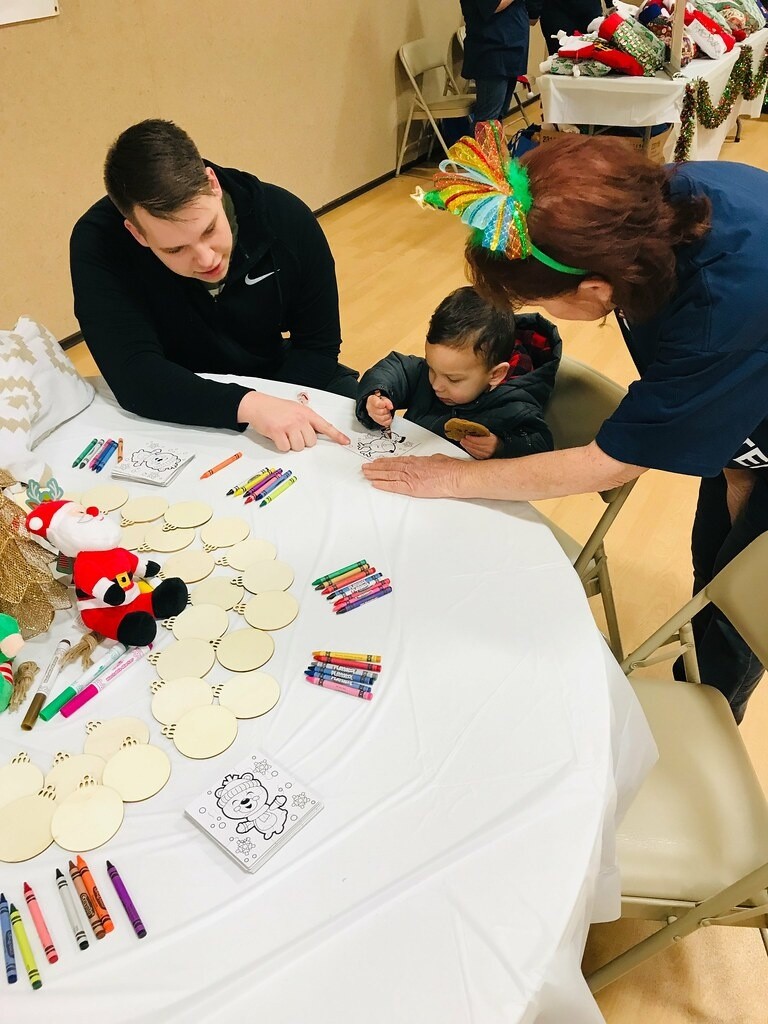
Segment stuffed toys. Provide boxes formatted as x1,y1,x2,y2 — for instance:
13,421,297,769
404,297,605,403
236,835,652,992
26,500,188,647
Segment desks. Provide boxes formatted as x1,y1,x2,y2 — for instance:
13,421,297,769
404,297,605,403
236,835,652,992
536,27,768,161
0,369,660,1023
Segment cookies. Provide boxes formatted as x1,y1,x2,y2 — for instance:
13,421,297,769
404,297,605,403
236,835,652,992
445,418,490,441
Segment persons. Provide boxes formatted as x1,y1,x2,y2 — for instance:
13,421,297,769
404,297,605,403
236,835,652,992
459,0,529,138
360,127,768,725
526,0,617,56
69,119,360,452
356,287,562,458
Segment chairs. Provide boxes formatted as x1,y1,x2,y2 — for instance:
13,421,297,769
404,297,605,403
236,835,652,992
586,530,768,995
540,356,636,662
394,38,476,178
457,24,536,144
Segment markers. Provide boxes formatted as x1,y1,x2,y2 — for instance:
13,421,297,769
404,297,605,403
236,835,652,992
305,651,382,700
118,437,123,463
255,470,292,501
199,452,242,479
56,868,91,950
38,641,131,720
312,559,393,615
258,476,298,507
69,855,114,940
374,389,392,438
22,882,60,964
9,905,44,991
225,467,284,504
60,642,153,718
106,860,148,940
21,639,72,731
1,893,17,985
71,439,118,473
